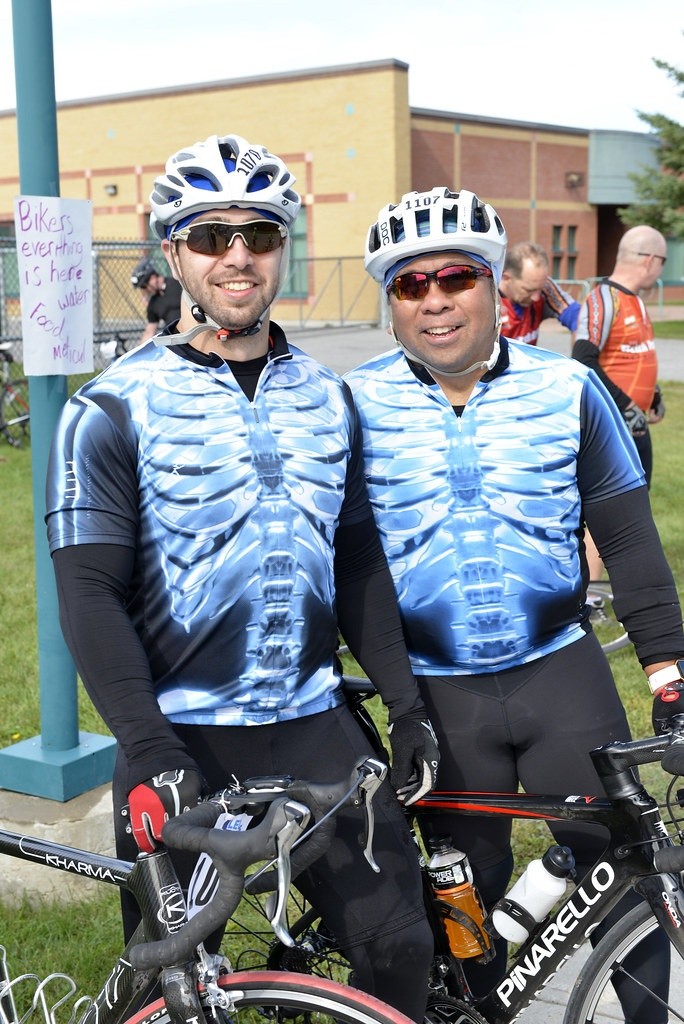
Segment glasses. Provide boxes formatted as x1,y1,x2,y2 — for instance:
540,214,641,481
385,262,493,300
638,253,667,266
170,220,287,255
140,276,151,290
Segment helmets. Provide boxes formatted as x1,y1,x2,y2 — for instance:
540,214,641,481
149,133,302,241
363,186,508,281
131,263,154,289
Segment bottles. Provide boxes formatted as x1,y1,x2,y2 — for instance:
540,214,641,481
492,846,573,943
428,836,488,957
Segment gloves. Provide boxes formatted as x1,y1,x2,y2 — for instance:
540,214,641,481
387,713,440,806
127,768,212,854
652,682,684,735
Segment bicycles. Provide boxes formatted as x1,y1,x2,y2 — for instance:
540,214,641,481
94,334,131,362
0,340,31,452
0,678,684,1024
335,571,631,659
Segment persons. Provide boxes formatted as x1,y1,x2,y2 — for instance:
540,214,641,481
43,136,441,1024
131,262,182,345
343,186,684,1024
579,224,669,627
491,241,580,355
